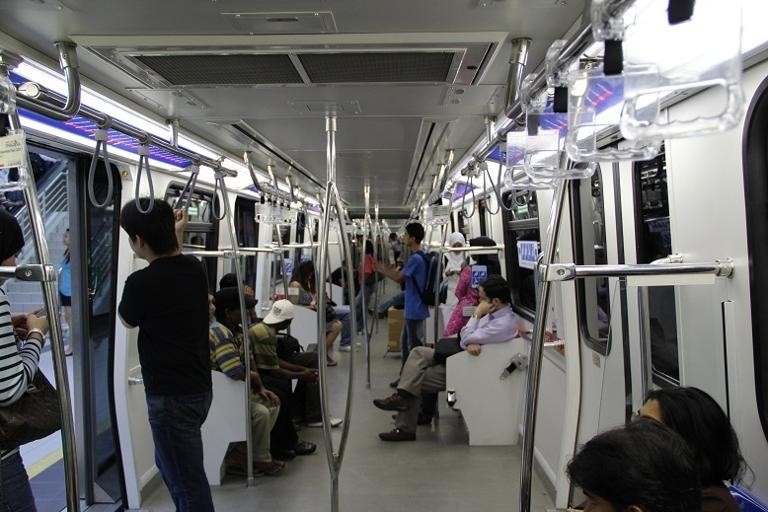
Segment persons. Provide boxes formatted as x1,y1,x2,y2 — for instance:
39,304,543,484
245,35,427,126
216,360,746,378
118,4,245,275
207,272,342,477
370,223,431,388
58,227,71,358
294,261,342,366
0,191,24,210
0,209,49,512
117,196,212,512
437,233,502,336
634,386,755,511
373,276,515,442
325,232,402,352
561,415,703,511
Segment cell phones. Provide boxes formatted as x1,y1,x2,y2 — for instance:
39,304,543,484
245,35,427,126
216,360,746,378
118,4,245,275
15,307,61,340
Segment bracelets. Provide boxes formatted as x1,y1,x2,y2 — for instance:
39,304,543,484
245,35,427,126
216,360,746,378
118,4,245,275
25,328,47,348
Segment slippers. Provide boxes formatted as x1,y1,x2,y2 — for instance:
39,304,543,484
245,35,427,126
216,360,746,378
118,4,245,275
226,455,286,479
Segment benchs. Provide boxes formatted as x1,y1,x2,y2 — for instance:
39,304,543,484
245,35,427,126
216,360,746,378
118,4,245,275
419,302,528,449
179,279,348,492
724,482,766,512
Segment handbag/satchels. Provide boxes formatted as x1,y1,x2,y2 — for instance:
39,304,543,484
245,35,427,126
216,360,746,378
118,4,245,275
434,337,465,366
0,363,63,450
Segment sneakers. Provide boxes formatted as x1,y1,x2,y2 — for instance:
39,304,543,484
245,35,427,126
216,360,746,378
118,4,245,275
338,341,362,352
326,354,337,366
391,412,433,425
370,392,412,412
271,436,318,462
63,344,73,357
378,424,418,442
308,414,344,430
388,378,400,388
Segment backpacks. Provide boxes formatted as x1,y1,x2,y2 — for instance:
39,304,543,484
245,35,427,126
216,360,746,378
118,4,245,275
421,249,449,307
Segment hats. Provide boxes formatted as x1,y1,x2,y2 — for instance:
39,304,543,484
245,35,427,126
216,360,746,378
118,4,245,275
220,273,249,289
262,299,295,325
214,286,258,312
